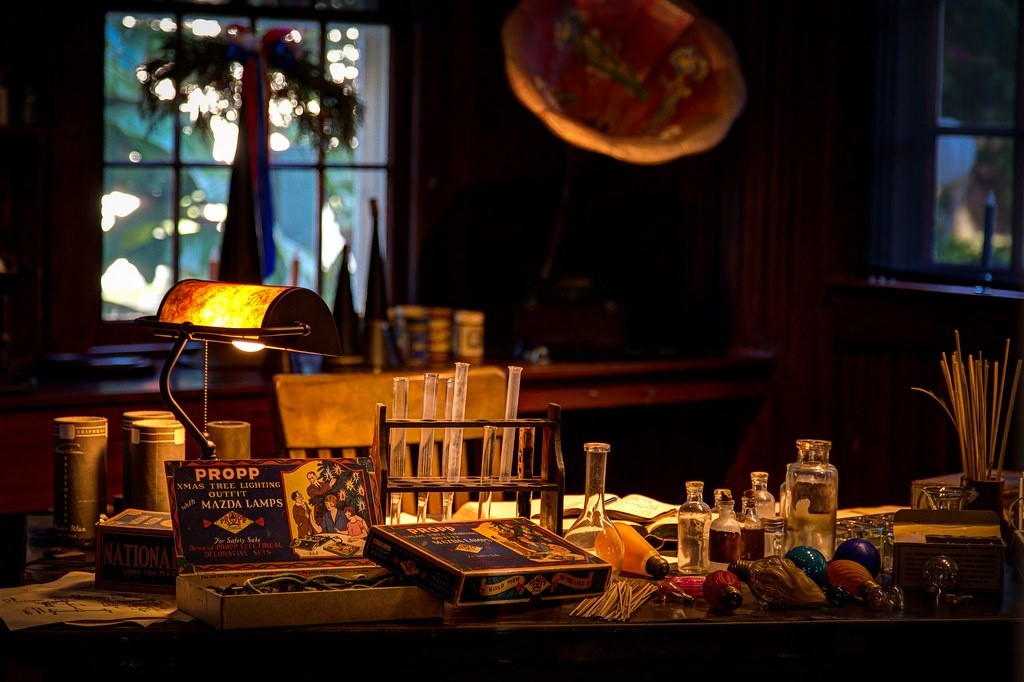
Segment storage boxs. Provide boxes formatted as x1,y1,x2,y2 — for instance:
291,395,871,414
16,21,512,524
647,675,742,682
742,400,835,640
889,508,1007,605
176,569,444,627
359,518,614,608
95,508,181,594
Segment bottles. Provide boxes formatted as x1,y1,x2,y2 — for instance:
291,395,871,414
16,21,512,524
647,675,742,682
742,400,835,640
741,472,776,521
738,497,765,562
779,463,792,518
564,442,625,576
784,439,839,564
922,485,966,511
710,488,737,522
678,481,711,576
707,499,740,574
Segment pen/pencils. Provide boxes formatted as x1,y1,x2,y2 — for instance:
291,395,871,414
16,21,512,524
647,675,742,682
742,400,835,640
980,190,995,289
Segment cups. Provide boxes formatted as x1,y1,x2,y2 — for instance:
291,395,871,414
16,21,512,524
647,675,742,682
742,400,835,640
761,516,785,558
961,475,1006,519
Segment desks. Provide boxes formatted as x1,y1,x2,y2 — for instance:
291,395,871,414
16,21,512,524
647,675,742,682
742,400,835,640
0,501,1024,682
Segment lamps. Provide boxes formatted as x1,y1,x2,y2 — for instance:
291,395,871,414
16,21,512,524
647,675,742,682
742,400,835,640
153,279,336,465
